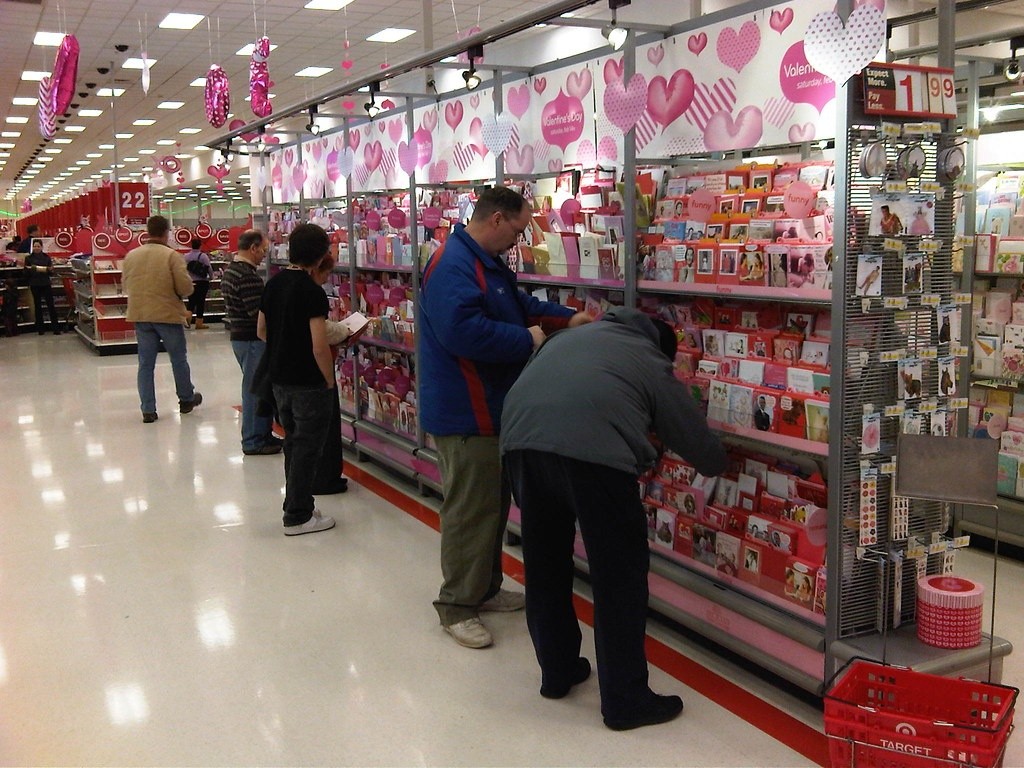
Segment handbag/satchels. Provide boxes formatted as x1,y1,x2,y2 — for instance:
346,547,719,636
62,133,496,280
186,253,211,278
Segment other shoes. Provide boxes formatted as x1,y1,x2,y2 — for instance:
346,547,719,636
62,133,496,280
311,478,348,495
54,331,61,335
38,329,45,335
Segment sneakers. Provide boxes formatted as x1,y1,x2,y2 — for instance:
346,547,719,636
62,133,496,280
180,393,202,414
284,508,335,536
443,617,493,649
143,411,158,423
481,588,527,611
243,443,281,455
540,656,591,699
268,434,285,446
605,690,683,730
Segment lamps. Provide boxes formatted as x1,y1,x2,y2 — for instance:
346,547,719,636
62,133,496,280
253,125,265,153
462,44,484,92
601,1,632,52
306,103,319,136
1003,35,1024,83
885,23,897,63
221,138,233,162
364,80,380,119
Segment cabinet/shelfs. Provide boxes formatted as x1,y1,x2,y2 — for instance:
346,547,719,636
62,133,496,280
0,0,1024,768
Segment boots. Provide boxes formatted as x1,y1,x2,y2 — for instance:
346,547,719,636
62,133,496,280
195,318,209,329
185,311,192,329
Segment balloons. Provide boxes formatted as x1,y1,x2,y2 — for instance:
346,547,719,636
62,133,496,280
341,360,411,394
783,181,815,219
338,282,404,305
366,208,442,229
560,199,583,226
688,188,716,221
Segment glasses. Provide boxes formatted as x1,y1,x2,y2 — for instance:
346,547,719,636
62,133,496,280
494,210,523,242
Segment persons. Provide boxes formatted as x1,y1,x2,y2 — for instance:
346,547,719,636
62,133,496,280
18,225,40,254
497,304,729,731
222,229,284,455
772,253,815,288
784,569,812,601
880,205,903,234
739,253,764,280
23,241,62,336
182,239,214,329
121,215,202,423
678,248,694,283
418,186,594,650
255,222,355,535
755,396,770,431
745,552,757,572
6,236,22,251
723,254,735,273
858,266,880,295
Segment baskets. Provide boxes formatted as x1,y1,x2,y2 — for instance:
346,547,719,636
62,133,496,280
818,655,1020,768
